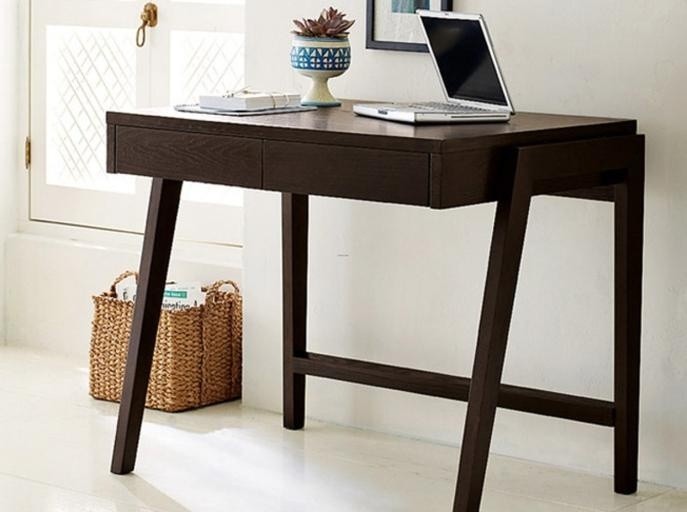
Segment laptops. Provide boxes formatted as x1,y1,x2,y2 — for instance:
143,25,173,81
353,10,516,124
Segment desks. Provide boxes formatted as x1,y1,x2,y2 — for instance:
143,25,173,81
105,96,645,512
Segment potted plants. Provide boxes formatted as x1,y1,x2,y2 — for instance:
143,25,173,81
288,7,355,106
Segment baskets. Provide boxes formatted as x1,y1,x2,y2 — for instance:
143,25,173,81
88,271,242,412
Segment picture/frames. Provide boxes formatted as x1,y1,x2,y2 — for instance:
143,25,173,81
365,0,453,52
